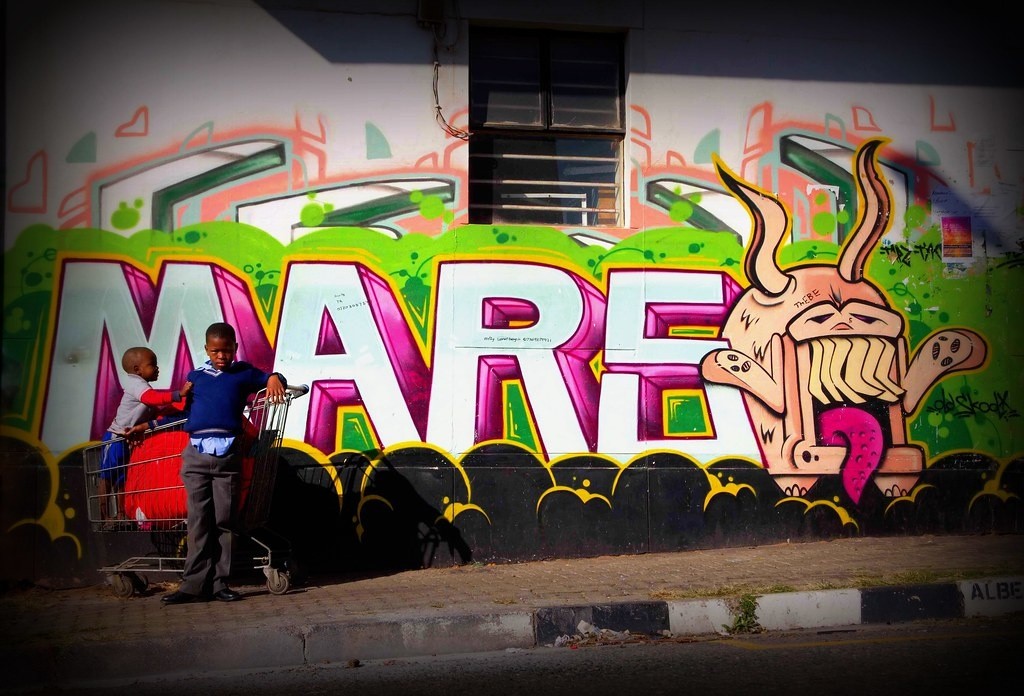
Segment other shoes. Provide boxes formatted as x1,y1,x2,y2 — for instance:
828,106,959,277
100,514,135,531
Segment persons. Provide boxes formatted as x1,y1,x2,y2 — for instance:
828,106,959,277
98,346,193,529
124,323,287,605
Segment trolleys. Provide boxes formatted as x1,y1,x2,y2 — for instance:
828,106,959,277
81,383,310,597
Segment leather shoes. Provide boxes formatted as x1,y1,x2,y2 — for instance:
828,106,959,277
162,588,198,603
214,590,239,603
